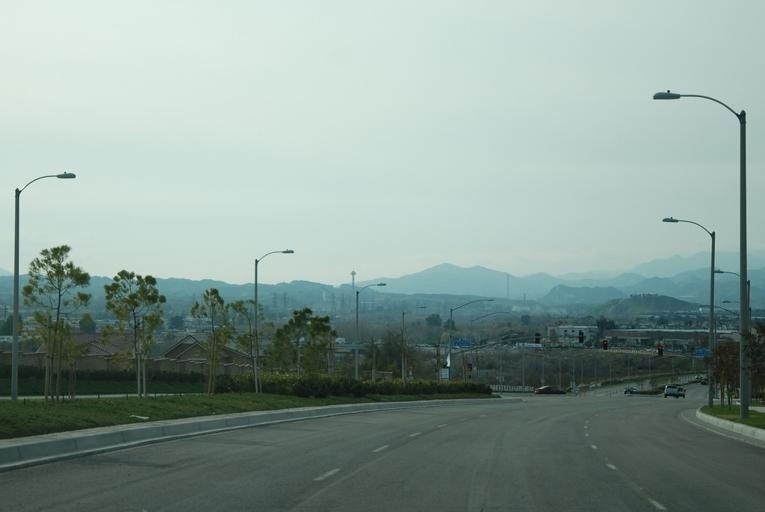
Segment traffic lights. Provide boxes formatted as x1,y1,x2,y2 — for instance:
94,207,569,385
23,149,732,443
657,344,663,357
602,339,608,351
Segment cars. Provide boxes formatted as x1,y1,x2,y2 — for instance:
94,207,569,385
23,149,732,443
533,385,566,395
619,373,711,399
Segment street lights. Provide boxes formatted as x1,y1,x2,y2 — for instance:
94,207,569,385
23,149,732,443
399,305,428,383
468,312,511,364
252,249,297,373
651,87,753,423
8,171,77,401
447,297,495,380
352,282,388,379
660,215,753,409
